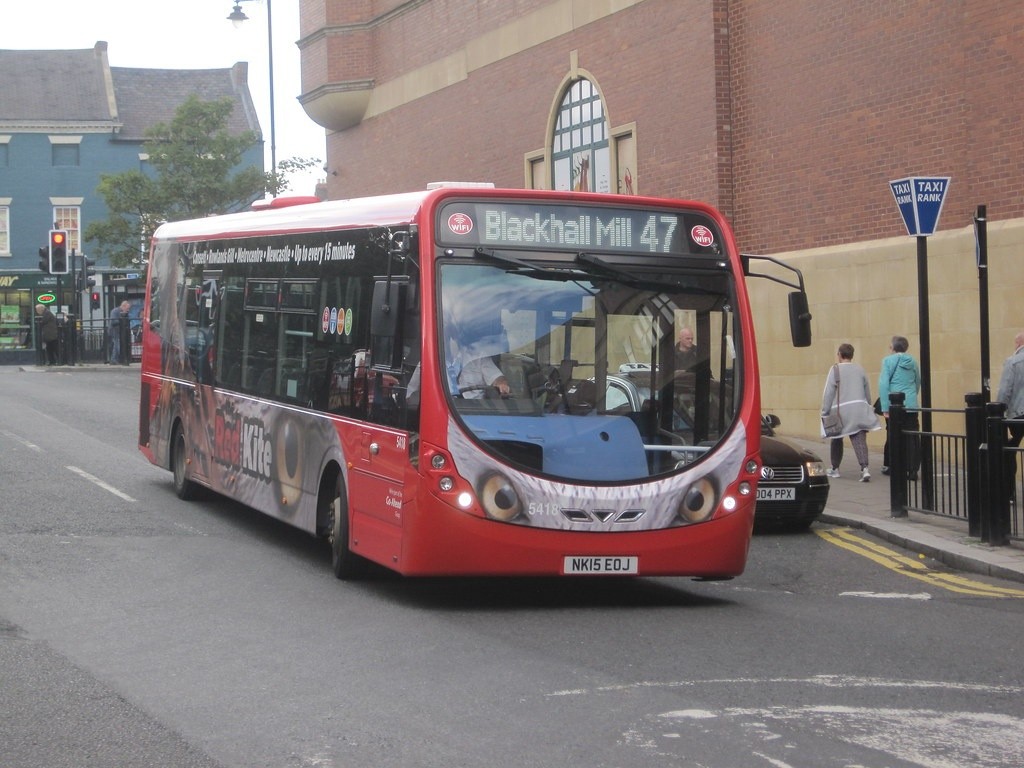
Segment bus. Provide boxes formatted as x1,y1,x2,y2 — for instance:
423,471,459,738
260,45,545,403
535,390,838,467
139,181,812,582
0,267,148,351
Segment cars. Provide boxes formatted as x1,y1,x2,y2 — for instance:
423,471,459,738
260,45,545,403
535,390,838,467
561,362,830,529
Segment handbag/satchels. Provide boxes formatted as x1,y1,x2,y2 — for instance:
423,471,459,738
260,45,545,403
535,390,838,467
874,392,905,416
822,413,843,437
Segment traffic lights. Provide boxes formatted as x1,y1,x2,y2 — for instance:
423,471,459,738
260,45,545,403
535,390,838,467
48,230,69,274
91,291,100,309
83,255,96,289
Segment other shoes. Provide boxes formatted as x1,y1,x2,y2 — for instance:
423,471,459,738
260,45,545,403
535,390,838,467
881,467,891,476
907,472,918,481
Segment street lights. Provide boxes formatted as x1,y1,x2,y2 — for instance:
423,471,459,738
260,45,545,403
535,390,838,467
225,0,277,200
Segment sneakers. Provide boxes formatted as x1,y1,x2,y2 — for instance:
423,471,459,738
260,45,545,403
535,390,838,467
826,468,840,477
859,466,871,482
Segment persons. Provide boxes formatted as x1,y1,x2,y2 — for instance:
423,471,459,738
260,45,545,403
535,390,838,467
818,343,876,482
33,304,59,366
110,301,130,364
998,332,1024,503
133,308,146,342
405,314,510,413
877,335,922,481
